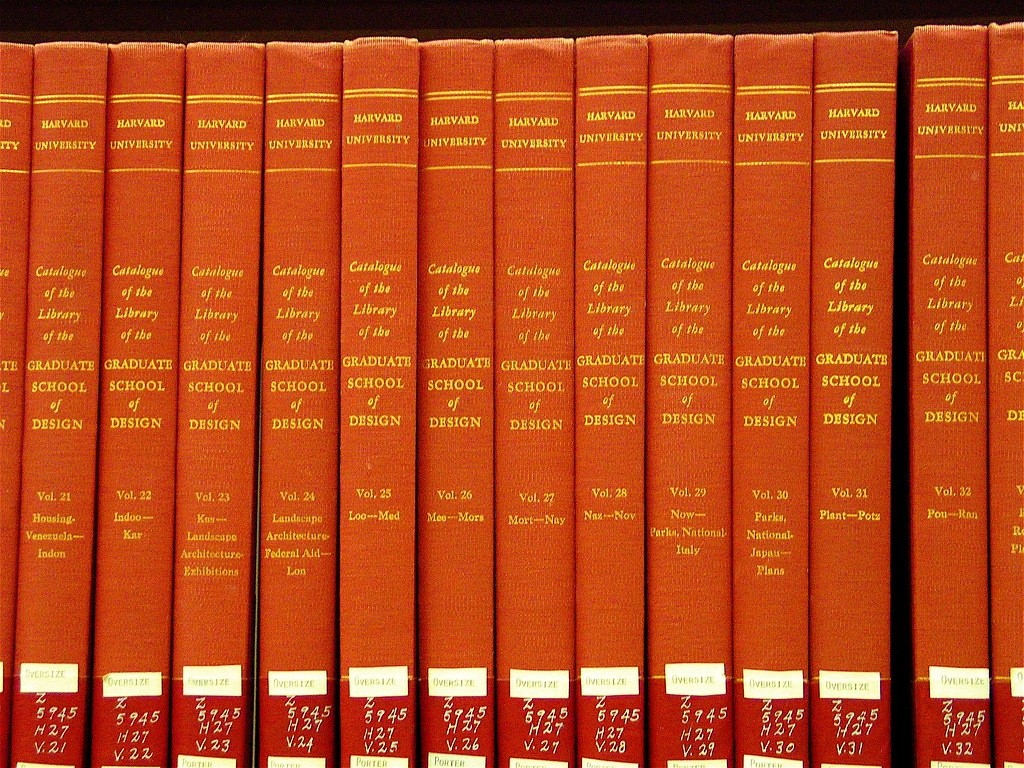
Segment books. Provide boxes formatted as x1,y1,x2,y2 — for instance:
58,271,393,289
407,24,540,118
1,22,1024,768
